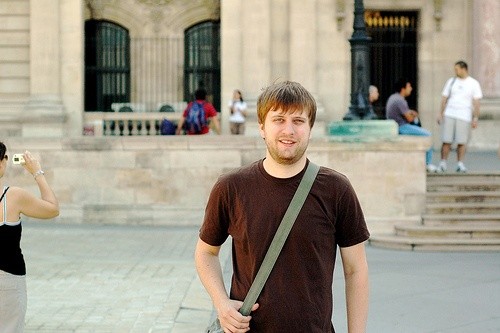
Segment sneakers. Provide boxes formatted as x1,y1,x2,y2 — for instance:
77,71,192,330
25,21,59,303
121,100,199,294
456,161,467,173
439,160,446,171
426,165,442,173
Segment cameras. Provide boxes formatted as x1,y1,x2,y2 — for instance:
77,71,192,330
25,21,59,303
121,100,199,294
13,153,25,164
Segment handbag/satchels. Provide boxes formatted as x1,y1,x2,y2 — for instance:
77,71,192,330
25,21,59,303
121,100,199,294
410,116,421,127
207,311,250,333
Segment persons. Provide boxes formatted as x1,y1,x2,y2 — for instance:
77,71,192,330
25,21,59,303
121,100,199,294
111,87,221,135
437,61,482,172
194,80,370,333
367,85,382,120
0,142,59,332
386,79,439,173
227,89,249,135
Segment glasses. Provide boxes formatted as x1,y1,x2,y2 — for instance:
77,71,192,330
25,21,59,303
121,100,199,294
2,155,8,160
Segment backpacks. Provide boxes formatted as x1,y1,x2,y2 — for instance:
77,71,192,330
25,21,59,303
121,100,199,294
160,120,176,135
185,100,207,132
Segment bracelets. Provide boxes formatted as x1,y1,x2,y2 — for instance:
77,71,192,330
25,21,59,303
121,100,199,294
32,169,45,179
